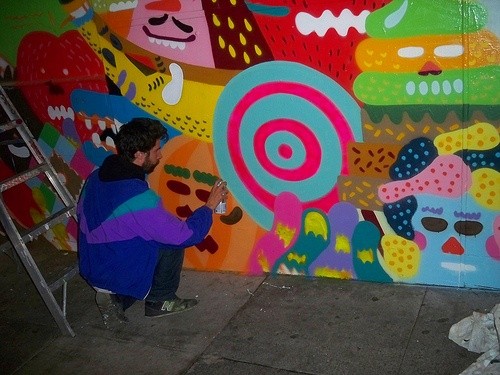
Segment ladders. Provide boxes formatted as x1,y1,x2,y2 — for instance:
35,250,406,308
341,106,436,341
0,83,77,338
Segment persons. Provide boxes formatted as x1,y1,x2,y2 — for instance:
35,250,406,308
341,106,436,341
78,118,229,331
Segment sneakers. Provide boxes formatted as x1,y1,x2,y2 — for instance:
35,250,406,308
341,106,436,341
96,292,128,333
144,296,200,319
